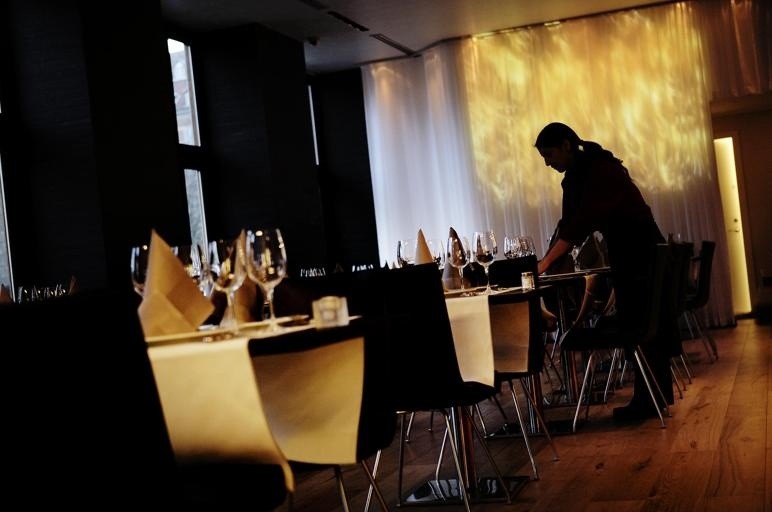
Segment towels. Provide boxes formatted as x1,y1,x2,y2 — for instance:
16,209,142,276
136,228,215,337
414,229,433,264
441,226,466,288
545,219,574,274
576,231,604,268
222,230,257,324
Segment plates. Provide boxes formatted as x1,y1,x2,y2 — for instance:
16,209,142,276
477,283,506,293
238,312,311,332
439,288,476,299
143,322,232,350
584,264,614,274
535,268,588,280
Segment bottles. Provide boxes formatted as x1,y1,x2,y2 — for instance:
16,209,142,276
521,271,535,293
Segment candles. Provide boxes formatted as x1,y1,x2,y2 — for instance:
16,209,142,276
445,285,553,387
143,315,370,493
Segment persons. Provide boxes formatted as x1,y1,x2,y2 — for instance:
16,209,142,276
534,123,675,419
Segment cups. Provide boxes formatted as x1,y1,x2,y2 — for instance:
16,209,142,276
12,282,68,306
472,231,499,295
209,238,248,324
299,265,329,278
448,234,472,297
428,236,445,272
246,228,288,320
502,231,540,258
173,243,204,287
347,263,379,272
394,238,416,268
130,242,151,295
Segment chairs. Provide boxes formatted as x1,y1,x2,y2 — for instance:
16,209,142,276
0,293,286,512
338,263,513,512
556,243,672,433
272,267,399,512
655,239,722,401
462,256,560,481
